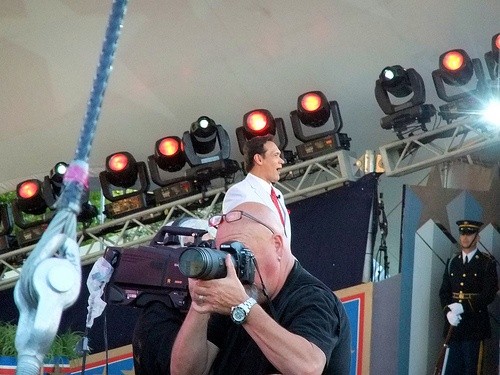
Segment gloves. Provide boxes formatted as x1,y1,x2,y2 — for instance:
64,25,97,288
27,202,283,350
448,303,464,315
447,311,462,326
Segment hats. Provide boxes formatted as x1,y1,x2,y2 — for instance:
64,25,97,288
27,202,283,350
456,220,484,232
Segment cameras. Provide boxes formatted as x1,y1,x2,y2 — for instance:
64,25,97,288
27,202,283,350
178,241,255,286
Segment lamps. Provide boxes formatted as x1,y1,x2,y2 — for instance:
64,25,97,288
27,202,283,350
16,33,500,246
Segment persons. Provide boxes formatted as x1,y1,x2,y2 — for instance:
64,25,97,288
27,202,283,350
221,133,291,254
438,219,500,375
132,200,352,375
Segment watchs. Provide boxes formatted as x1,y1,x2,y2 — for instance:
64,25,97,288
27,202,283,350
230,297,257,325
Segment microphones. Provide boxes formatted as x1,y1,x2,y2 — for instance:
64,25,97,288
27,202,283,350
277,195,280,198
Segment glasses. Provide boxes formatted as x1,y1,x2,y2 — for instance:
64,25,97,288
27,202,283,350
208,210,275,234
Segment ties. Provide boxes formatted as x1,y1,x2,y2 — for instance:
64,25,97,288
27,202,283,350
465,255,469,266
270,188,285,226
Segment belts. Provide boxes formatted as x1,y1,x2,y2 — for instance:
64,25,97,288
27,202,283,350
452,293,480,299
